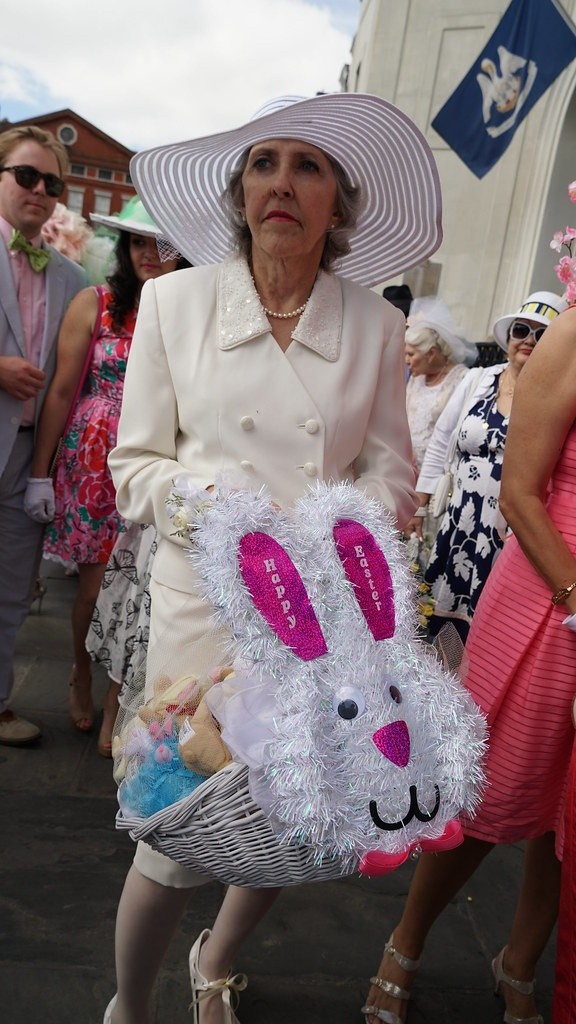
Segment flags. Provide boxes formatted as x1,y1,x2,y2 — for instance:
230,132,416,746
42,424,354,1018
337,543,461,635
431,0,576,178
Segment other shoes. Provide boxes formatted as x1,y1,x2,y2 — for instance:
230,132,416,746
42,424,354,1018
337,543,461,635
0,709,40,743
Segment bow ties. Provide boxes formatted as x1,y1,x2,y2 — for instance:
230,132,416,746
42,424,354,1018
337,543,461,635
7,229,51,271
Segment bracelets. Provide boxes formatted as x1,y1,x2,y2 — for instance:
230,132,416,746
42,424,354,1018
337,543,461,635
552,582,576,605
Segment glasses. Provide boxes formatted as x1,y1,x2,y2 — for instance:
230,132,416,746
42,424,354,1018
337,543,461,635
509,322,548,344
0,165,66,197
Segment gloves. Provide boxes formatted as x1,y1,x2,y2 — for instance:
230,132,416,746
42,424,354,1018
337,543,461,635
24,478,55,522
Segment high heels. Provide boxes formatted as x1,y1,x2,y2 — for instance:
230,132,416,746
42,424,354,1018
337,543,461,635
361,932,423,1024
492,945,543,1024
188,927,249,1024
104,992,119,1024
98,692,117,757
68,671,96,729
32,577,46,615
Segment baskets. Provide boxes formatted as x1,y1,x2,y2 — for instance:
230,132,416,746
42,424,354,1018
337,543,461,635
113,759,362,889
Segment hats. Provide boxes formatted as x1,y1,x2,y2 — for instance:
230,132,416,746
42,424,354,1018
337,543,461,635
491,291,569,353
381,284,413,306
129,92,442,288
90,192,168,240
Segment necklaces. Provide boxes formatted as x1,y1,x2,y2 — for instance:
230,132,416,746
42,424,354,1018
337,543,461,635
252,276,310,318
485,372,509,451
427,366,446,384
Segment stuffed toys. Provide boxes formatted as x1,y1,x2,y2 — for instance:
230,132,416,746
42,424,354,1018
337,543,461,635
112,665,241,822
203,488,490,853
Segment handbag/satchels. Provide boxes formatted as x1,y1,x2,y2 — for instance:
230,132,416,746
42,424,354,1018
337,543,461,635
430,469,452,518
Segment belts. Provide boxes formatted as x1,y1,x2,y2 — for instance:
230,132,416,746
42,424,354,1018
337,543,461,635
17,424,34,435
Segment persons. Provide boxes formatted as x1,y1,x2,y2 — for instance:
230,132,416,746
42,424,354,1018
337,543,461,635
403,292,569,647
0,126,92,746
23,202,195,756
106,91,445,1024
382,286,472,574
362,300,576,1024
